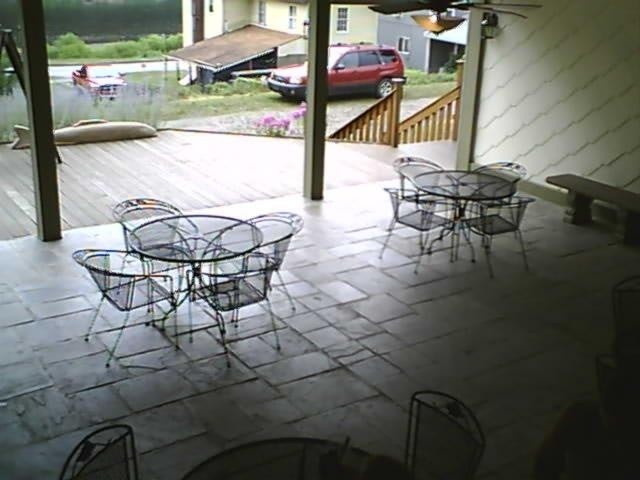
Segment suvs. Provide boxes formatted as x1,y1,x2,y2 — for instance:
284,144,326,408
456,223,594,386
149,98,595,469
267,43,408,102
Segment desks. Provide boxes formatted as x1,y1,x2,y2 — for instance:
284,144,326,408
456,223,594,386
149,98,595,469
179,435,419,480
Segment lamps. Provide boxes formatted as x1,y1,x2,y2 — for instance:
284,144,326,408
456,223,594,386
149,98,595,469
410,9,465,36
481,13,499,39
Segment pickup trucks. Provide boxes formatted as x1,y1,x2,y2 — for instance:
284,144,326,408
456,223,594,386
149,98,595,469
69,63,131,101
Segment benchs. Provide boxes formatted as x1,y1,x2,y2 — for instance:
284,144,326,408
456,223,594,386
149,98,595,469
546,174,639,247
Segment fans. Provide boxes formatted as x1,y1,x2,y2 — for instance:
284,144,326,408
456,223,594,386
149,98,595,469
330,0,543,20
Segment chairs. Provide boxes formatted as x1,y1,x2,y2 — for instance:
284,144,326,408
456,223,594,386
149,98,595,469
57,423,142,479
403,391,487,479
72,197,304,369
378,157,536,278
